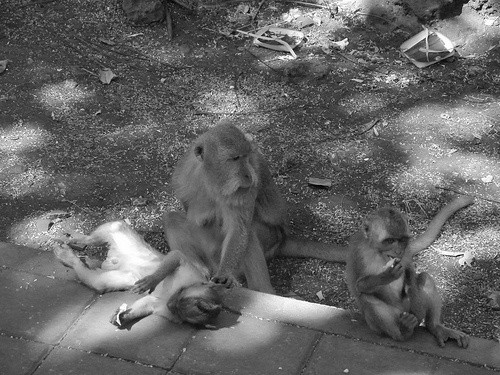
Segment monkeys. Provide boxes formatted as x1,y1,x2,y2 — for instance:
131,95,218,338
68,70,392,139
52,222,222,331
345,205,471,349
160,120,475,296
